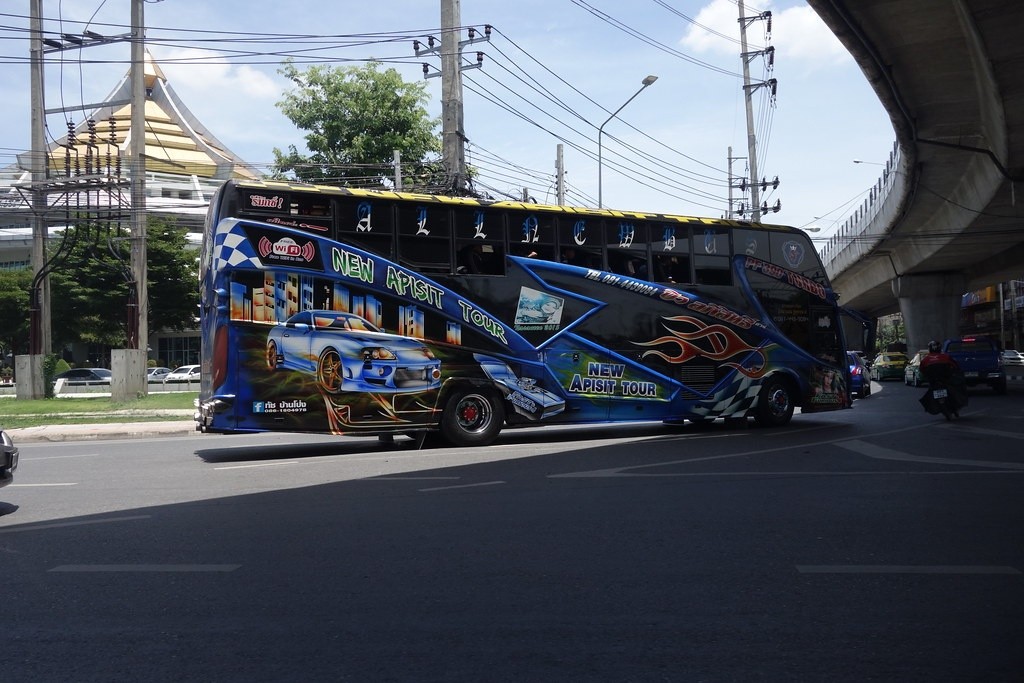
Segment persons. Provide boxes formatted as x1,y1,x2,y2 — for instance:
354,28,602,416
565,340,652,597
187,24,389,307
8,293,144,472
541,298,560,314
920,340,969,409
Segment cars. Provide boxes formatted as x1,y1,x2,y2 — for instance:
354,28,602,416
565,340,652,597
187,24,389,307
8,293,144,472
0,431,19,490
904,350,952,387
872,352,912,382
166,364,202,382
998,349,1024,367
846,350,872,399
852,350,871,371
51,366,112,386
146,366,174,384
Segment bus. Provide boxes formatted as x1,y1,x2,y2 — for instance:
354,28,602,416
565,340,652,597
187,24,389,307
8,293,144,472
190,176,854,449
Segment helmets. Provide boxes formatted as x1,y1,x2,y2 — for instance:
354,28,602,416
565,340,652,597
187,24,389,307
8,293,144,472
928,340,942,352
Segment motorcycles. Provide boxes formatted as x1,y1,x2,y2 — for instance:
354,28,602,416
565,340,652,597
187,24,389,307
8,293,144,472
918,368,969,421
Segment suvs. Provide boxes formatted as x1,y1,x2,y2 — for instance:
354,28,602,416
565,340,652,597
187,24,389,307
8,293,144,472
943,336,1005,392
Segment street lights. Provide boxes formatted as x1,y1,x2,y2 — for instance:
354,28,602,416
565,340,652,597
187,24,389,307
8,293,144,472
597,75,659,209
